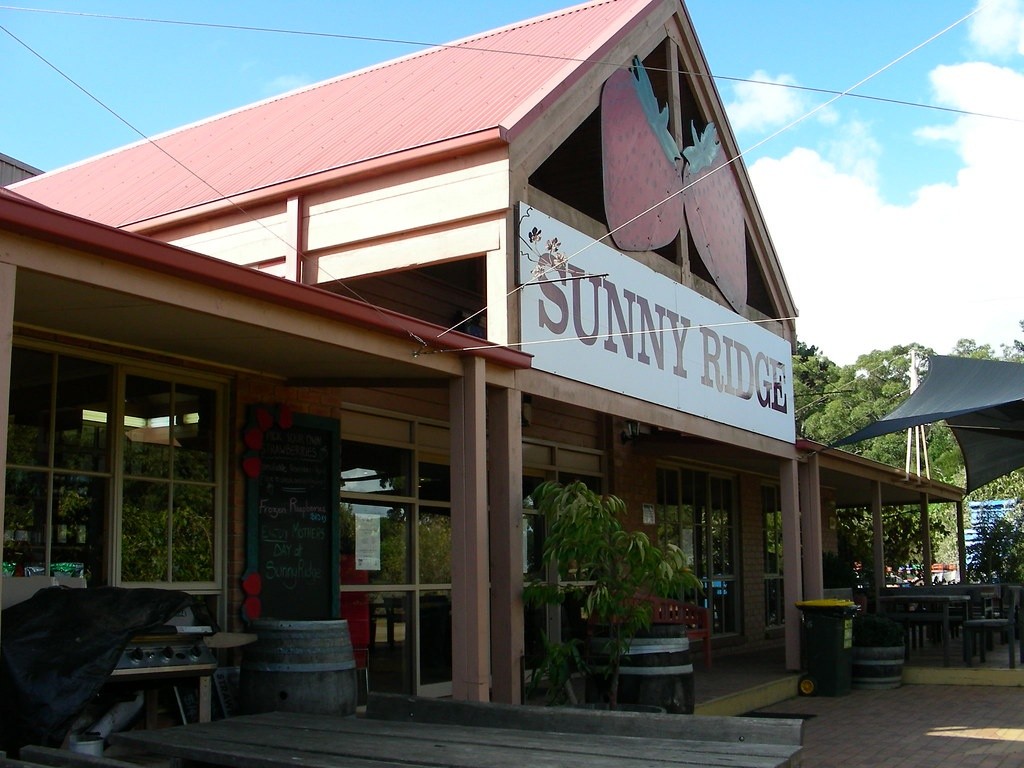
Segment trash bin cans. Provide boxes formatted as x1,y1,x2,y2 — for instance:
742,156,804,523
796,603,861,696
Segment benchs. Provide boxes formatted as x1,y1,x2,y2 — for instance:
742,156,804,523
0,742,136,768
365,691,805,746
610,591,711,670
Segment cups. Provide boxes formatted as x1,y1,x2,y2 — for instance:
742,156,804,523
57,524,67,543
75,525,87,544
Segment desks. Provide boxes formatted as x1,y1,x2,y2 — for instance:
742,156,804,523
106,709,804,768
879,593,971,667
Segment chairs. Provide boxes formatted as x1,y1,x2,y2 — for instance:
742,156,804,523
880,585,1024,667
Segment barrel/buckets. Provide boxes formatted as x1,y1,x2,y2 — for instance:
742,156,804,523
584,622,694,715
251,618,358,719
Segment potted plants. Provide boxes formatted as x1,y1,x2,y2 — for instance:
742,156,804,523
823,550,857,601
850,615,906,689
